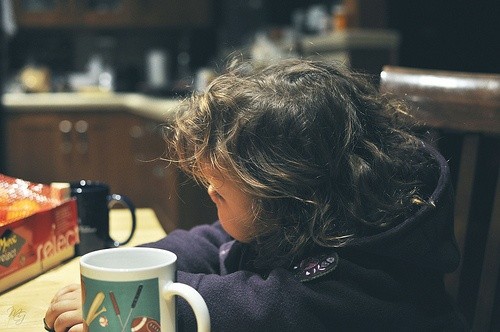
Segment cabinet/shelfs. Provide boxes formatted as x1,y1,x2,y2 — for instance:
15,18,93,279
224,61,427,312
2,106,220,235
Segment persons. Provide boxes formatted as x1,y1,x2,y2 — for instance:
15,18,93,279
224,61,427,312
43,52,500,332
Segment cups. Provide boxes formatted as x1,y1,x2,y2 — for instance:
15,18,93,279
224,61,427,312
69,181,135,258
79,248,211,332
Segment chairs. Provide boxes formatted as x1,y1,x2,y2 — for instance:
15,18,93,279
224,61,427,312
375,65,500,332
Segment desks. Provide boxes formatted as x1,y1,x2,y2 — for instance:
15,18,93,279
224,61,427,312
1,204,167,332
